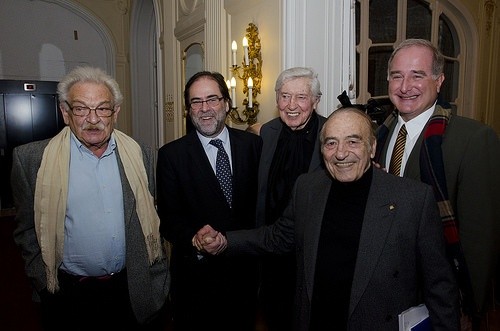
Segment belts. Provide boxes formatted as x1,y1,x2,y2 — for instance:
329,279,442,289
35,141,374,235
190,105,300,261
79,269,127,284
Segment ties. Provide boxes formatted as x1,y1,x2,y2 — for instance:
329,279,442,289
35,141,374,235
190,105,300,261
209,138,233,211
389,123,408,177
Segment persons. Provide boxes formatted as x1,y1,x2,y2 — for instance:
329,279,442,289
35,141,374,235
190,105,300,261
260,67,329,331
156,71,263,331
192,106,461,331
10,66,171,331
370,38,500,331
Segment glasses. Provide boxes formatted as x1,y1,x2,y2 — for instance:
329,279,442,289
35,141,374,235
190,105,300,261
65,99,115,118
189,95,224,110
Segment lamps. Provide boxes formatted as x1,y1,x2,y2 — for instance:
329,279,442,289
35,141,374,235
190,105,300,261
226,22,263,126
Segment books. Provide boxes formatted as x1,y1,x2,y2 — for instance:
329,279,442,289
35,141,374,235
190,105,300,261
399,303,432,331
